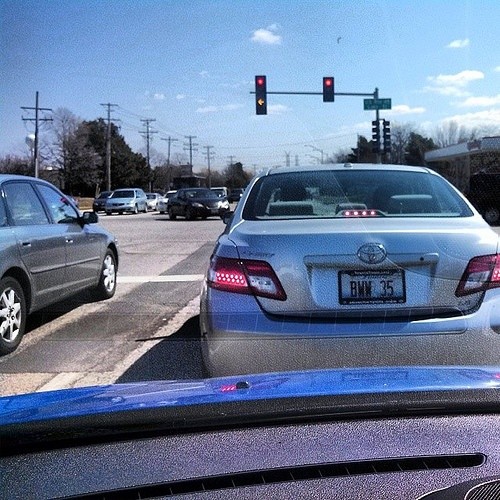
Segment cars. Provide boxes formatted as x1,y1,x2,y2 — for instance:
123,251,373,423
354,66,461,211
92,185,247,221
0,174,120,357
199,163,500,378
165,188,232,221
105,188,148,216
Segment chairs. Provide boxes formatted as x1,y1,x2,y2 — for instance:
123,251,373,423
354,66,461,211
269,201,315,217
335,203,368,214
383,195,436,215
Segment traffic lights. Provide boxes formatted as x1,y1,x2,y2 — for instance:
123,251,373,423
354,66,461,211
382,120,392,156
371,122,381,154
322,77,335,102
254,75,267,116
